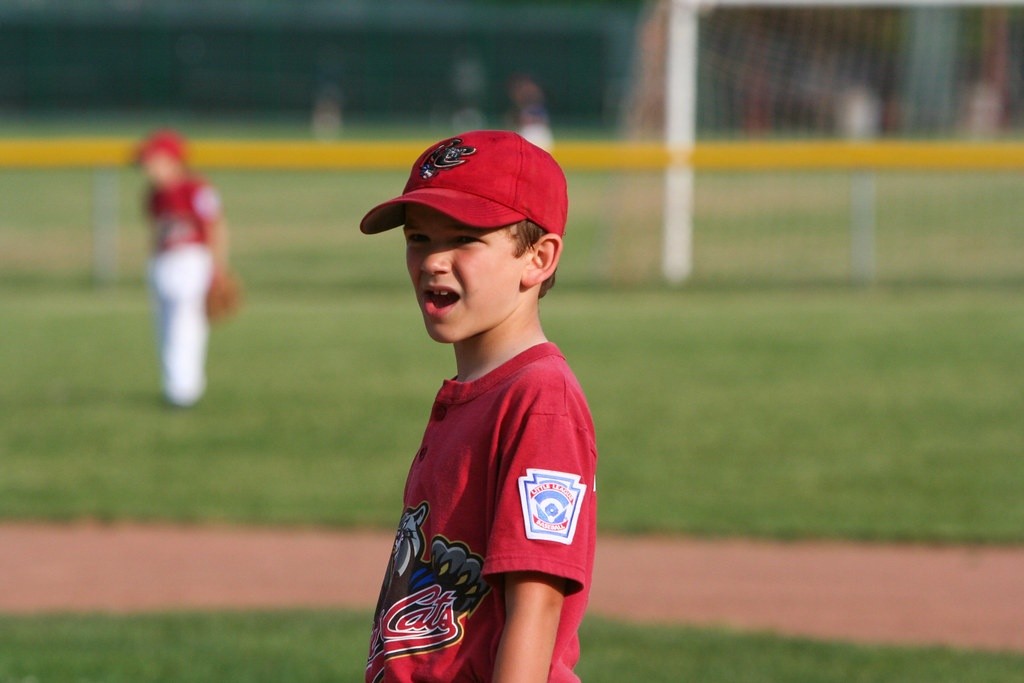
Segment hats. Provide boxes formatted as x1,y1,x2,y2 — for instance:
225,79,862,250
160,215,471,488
359,132,568,242
138,133,184,165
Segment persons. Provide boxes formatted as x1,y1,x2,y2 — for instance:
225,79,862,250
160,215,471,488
359,129,597,683
510,73,553,150
131,129,230,408
